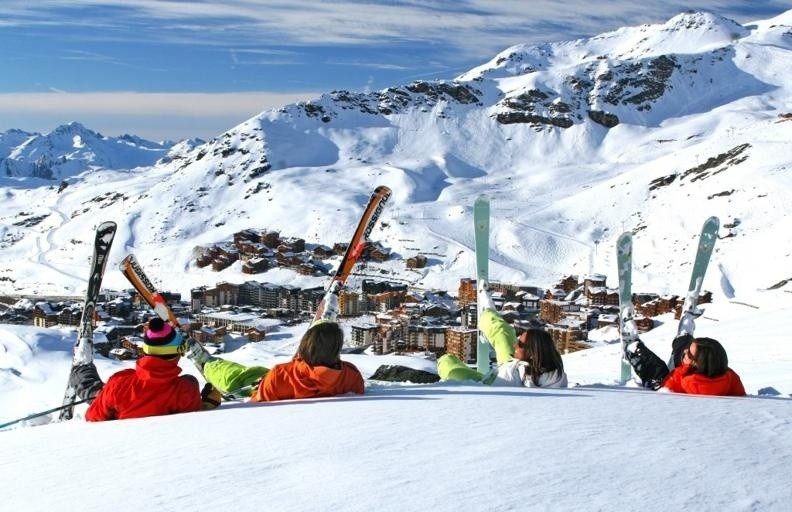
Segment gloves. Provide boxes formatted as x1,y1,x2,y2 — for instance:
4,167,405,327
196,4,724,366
201,383,221,410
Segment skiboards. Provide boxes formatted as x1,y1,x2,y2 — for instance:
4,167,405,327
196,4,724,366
616,217,720,383
372,198,490,384
118,185,392,400
59,222,116,421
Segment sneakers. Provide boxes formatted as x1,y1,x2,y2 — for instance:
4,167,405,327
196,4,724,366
184,337,210,372
624,320,638,341
479,289,494,310
330,282,341,296
74,337,94,365
679,319,695,338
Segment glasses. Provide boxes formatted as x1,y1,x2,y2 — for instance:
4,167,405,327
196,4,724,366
688,348,694,361
517,340,524,348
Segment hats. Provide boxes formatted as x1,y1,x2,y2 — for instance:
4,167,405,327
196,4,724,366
142,317,184,359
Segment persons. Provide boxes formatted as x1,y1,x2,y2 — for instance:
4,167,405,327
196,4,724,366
436,290,569,389
251,321,365,402
619,299,747,396
70,318,222,422
